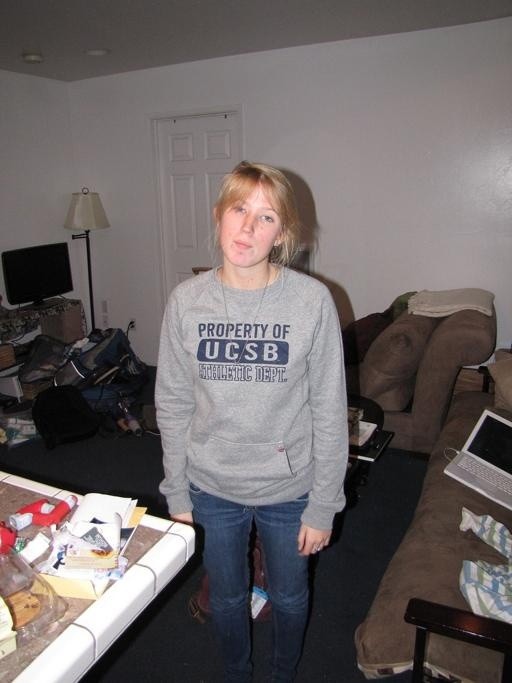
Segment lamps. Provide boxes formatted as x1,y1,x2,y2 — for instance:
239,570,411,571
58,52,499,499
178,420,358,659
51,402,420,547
129,318,136,330
64,187,110,330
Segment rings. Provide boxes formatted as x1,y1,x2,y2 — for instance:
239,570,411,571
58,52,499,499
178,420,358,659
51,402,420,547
316,546,321,551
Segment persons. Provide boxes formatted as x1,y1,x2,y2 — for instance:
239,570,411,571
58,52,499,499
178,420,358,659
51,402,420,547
152,155,352,680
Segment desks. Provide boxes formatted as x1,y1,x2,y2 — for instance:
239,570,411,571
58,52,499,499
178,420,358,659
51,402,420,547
0,471,196,683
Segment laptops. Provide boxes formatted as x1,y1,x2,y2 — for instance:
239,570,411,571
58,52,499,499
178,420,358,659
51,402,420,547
445,410,512,510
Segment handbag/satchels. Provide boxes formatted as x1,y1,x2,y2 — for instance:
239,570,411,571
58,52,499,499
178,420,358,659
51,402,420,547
18,327,140,387
31,385,98,448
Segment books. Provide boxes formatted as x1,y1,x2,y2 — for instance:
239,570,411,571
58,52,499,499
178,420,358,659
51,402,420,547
347,406,378,446
40,491,149,579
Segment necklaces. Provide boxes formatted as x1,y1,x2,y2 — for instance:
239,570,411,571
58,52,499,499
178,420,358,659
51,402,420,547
218,263,273,373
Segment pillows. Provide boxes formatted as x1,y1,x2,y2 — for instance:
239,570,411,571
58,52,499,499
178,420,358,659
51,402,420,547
342,291,433,412
487,356,512,405
493,349,512,412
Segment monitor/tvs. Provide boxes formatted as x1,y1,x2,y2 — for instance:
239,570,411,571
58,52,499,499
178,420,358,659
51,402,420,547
0,241,74,311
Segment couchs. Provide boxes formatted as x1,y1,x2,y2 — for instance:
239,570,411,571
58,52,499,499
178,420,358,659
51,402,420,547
353,366,512,683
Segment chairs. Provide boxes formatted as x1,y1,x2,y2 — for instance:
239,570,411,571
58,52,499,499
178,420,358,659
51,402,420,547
347,303,497,456
